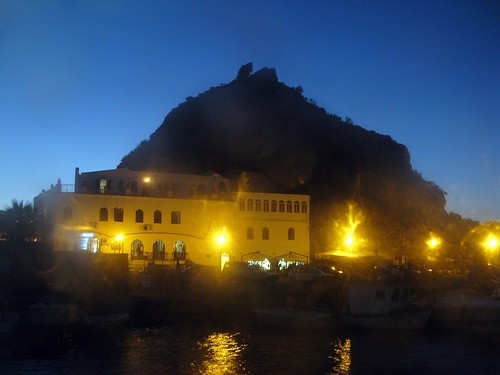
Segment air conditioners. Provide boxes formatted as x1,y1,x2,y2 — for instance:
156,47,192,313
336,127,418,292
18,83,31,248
143,226,150,231
101,238,109,245
